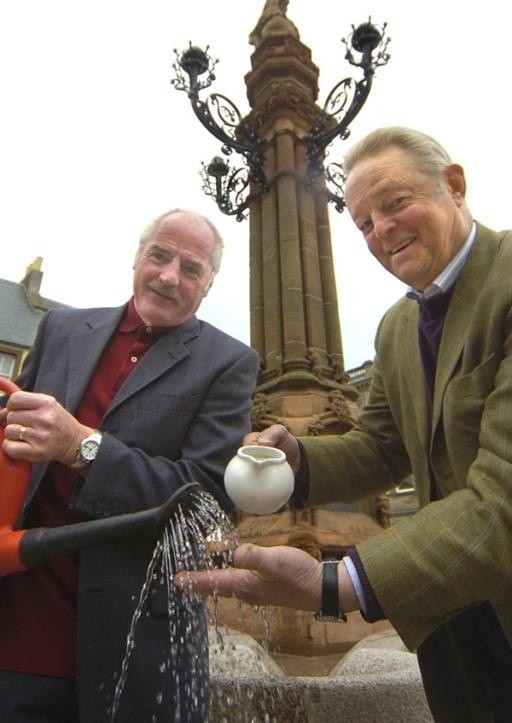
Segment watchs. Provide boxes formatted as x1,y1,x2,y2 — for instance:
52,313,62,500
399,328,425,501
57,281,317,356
69,428,103,471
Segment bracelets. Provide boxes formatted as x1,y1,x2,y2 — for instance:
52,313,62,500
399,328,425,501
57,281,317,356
312,558,349,622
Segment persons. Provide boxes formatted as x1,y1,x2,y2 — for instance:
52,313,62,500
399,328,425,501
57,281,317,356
172,123,512,723
2,206,263,723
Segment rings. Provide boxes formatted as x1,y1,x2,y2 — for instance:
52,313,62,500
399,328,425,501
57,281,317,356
19,425,29,440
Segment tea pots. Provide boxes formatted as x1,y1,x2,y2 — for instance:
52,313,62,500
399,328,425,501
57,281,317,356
222,443,297,518
